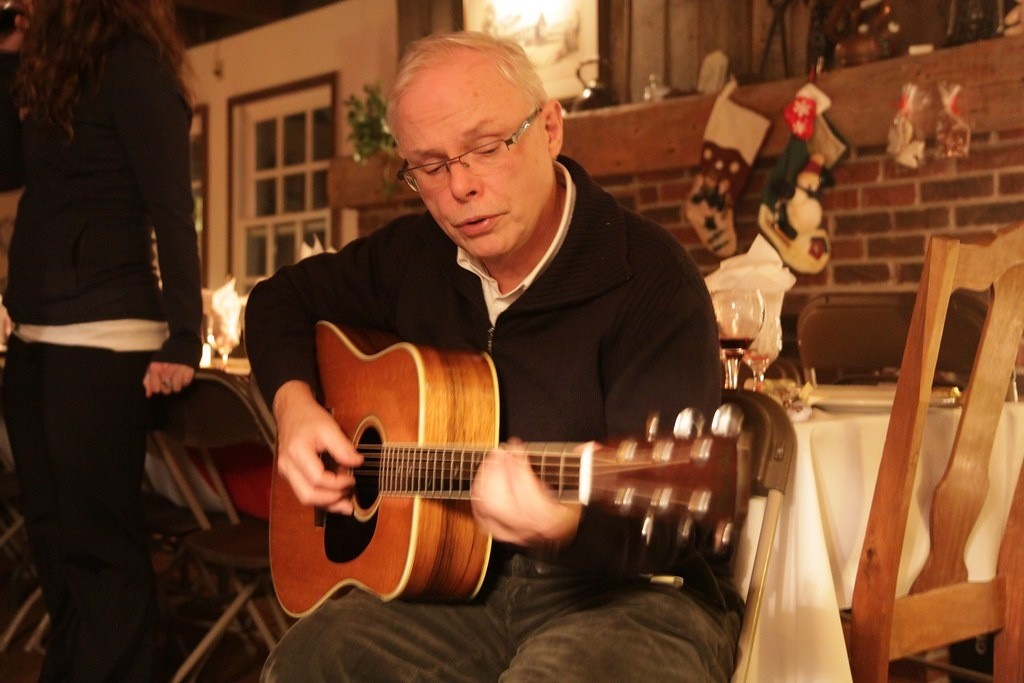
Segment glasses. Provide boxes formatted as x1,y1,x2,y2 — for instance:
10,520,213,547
396,101,547,193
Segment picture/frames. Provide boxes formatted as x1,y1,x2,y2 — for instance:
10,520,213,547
451,0,611,113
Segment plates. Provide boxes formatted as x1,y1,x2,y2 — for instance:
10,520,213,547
810,389,955,415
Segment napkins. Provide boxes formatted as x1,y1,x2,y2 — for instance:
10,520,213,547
704,233,796,356
201,278,243,347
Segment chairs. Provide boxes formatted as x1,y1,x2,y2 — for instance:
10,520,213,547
147,370,287,683
794,290,1020,403
842,220,1024,683
721,388,797,683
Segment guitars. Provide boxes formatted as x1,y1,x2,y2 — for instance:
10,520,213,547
268,319,758,619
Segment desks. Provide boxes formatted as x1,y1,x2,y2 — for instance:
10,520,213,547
207,355,1024,683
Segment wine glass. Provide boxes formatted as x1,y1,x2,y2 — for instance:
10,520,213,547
711,288,764,389
206,311,242,370
740,314,783,392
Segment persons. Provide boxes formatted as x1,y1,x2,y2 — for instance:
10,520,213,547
245,32,749,682
0,0,207,683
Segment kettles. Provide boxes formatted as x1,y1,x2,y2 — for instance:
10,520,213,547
570,59,622,111
